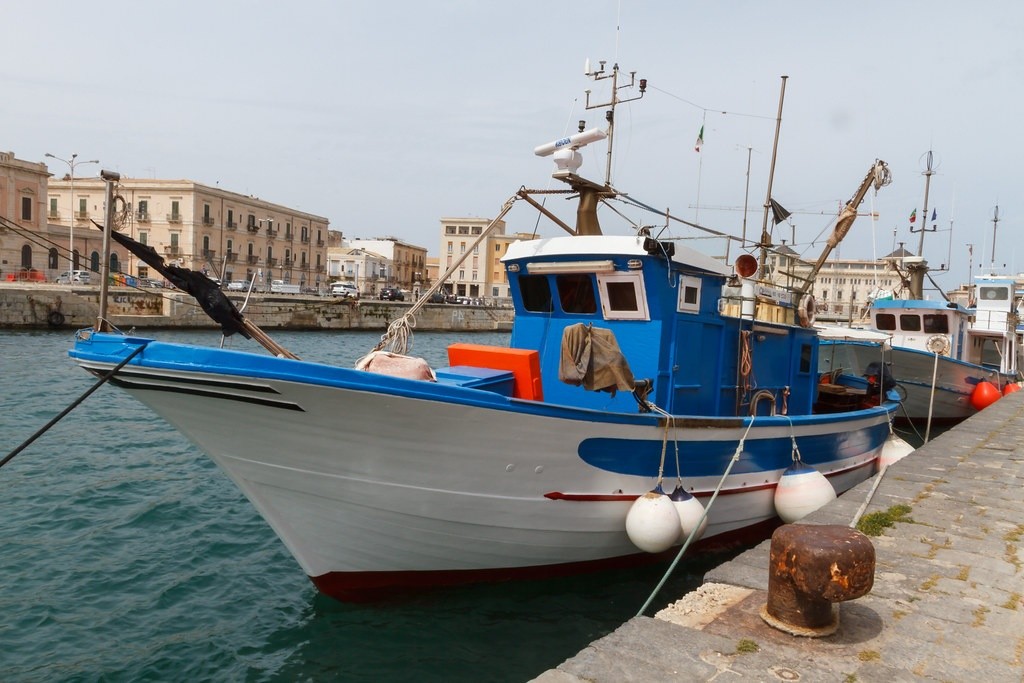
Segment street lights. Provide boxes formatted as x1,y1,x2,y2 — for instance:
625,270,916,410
44,153,100,285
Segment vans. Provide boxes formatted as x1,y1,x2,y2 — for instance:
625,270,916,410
330,281,360,299
55,270,91,285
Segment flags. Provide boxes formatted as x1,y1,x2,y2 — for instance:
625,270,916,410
932,208,937,220
909,207,916,223
695,123,703,151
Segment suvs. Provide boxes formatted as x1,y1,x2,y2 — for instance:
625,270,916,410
380,287,404,302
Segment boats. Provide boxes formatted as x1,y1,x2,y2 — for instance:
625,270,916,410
67,57,908,600
800,146,1024,421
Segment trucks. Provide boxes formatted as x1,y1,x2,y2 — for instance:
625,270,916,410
271,280,301,295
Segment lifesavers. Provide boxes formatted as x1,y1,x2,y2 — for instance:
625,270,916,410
47,311,65,326
797,294,816,327
926,334,950,355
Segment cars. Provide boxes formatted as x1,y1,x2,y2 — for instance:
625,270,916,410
149,281,163,288
138,277,151,287
421,290,444,304
227,280,257,293
